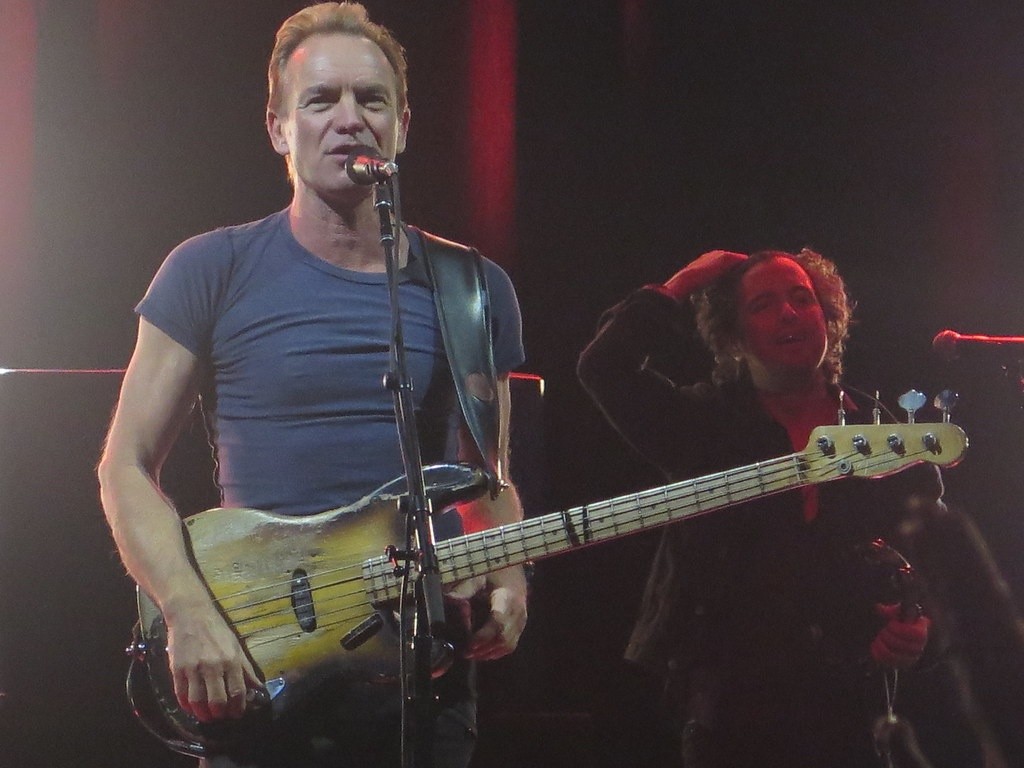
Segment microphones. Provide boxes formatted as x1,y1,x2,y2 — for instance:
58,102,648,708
933,330,1024,364
342,146,399,185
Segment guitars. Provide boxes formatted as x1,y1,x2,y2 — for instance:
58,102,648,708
130,382,976,763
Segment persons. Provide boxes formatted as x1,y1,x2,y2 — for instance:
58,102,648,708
98,0,529,768
575,246,943,767
